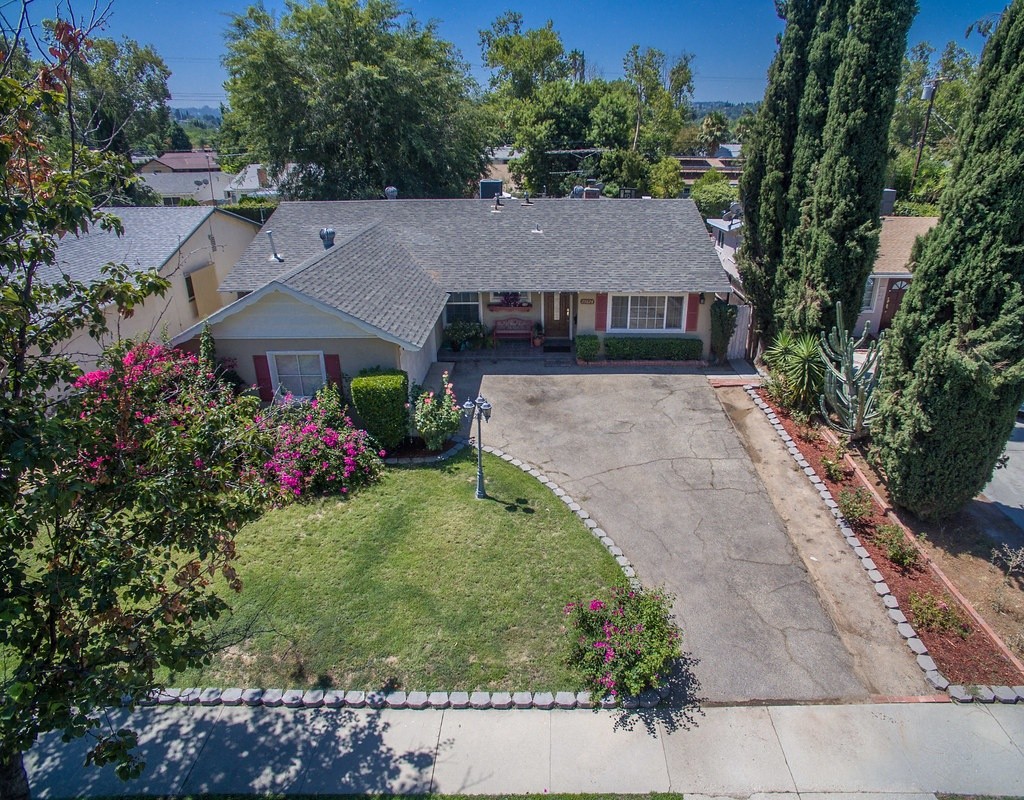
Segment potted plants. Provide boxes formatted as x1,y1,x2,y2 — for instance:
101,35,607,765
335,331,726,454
445,321,487,351
534,322,543,346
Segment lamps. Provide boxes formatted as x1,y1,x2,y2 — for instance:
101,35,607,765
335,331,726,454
699,293,705,304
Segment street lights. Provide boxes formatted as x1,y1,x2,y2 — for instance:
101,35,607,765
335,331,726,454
463,393,493,499
205,154,216,206
909,72,939,190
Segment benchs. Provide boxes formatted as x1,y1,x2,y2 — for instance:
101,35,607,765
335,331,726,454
493,318,533,348
